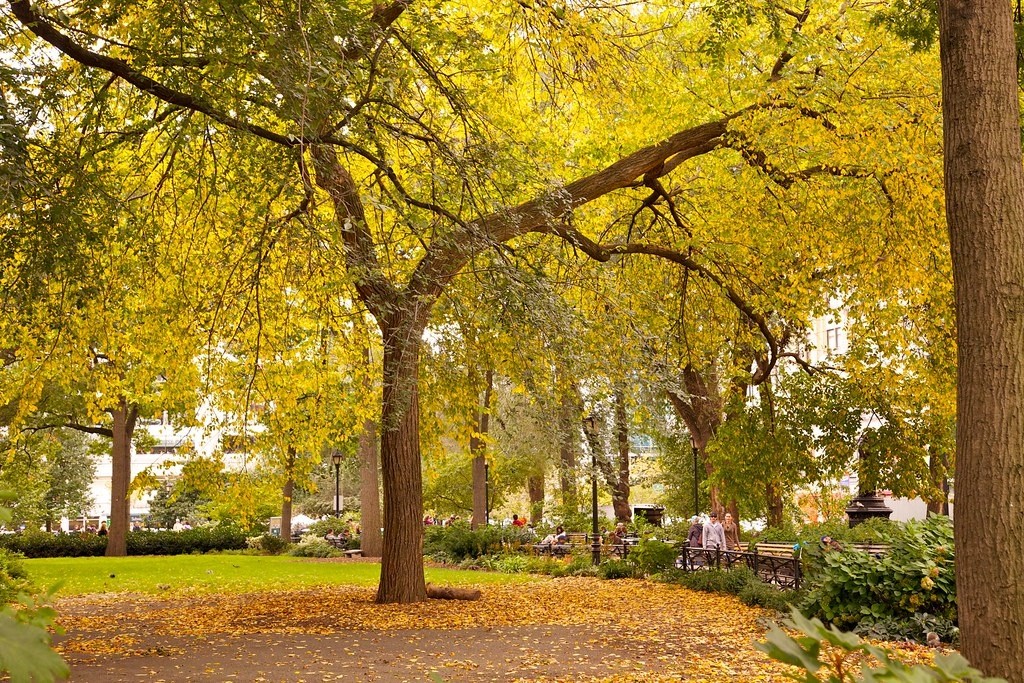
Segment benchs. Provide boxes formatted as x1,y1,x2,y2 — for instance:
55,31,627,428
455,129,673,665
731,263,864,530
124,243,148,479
752,542,797,559
564,533,587,544
733,542,750,566
841,543,892,562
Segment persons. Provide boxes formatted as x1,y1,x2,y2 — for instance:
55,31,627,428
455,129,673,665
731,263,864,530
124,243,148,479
598,521,631,555
324,518,362,550
423,512,474,532
485,510,569,558
720,512,744,571
819,535,846,557
129,516,194,532
702,512,727,568
688,515,705,565
0,519,112,537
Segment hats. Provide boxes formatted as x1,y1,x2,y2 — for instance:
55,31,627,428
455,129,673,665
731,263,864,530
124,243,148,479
711,513,717,516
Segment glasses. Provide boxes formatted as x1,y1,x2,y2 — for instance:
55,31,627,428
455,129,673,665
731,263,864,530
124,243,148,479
712,516,716,518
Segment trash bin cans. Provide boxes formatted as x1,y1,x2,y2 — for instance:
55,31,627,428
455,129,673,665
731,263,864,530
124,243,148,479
634,507,664,528
845,496,892,528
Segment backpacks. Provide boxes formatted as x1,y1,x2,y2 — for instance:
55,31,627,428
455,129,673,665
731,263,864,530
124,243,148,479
692,525,702,545
